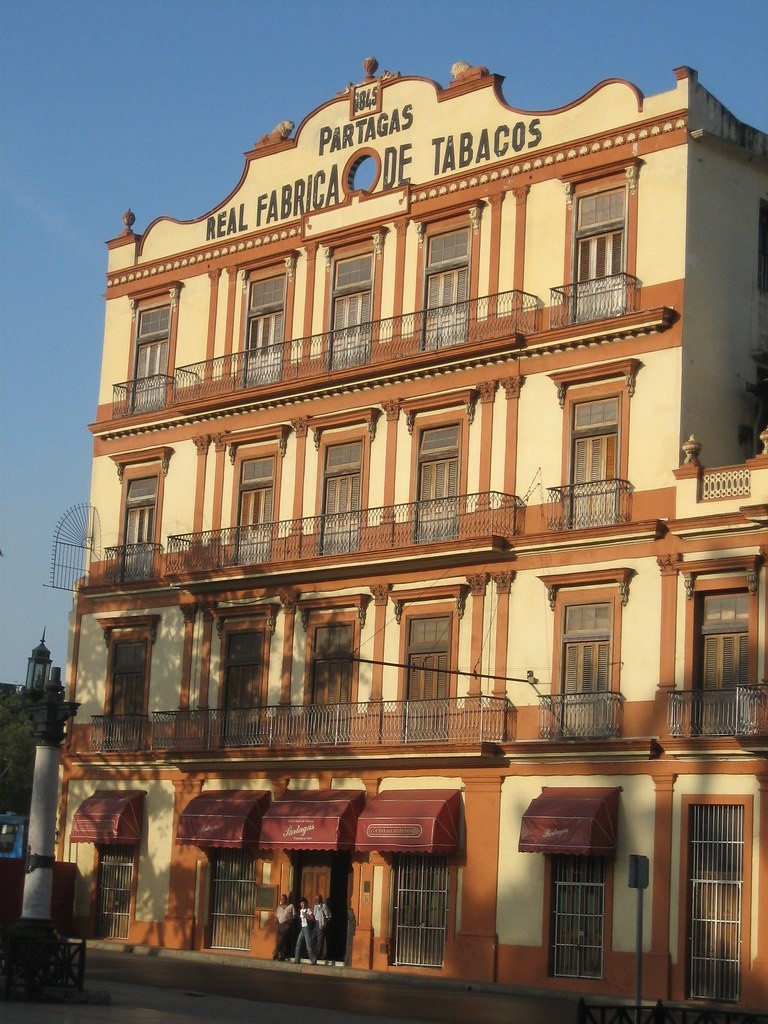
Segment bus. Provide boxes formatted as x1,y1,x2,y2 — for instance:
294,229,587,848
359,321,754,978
0,811,29,857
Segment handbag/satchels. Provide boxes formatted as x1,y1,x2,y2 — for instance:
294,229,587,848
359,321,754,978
306,907,316,929
321,902,327,926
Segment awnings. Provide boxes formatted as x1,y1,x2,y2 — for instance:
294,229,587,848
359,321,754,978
353,789,462,856
175,790,271,849
516,785,620,858
69,789,148,846
257,790,364,852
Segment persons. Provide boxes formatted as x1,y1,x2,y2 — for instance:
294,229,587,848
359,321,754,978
271,893,298,960
310,894,332,959
289,897,318,966
